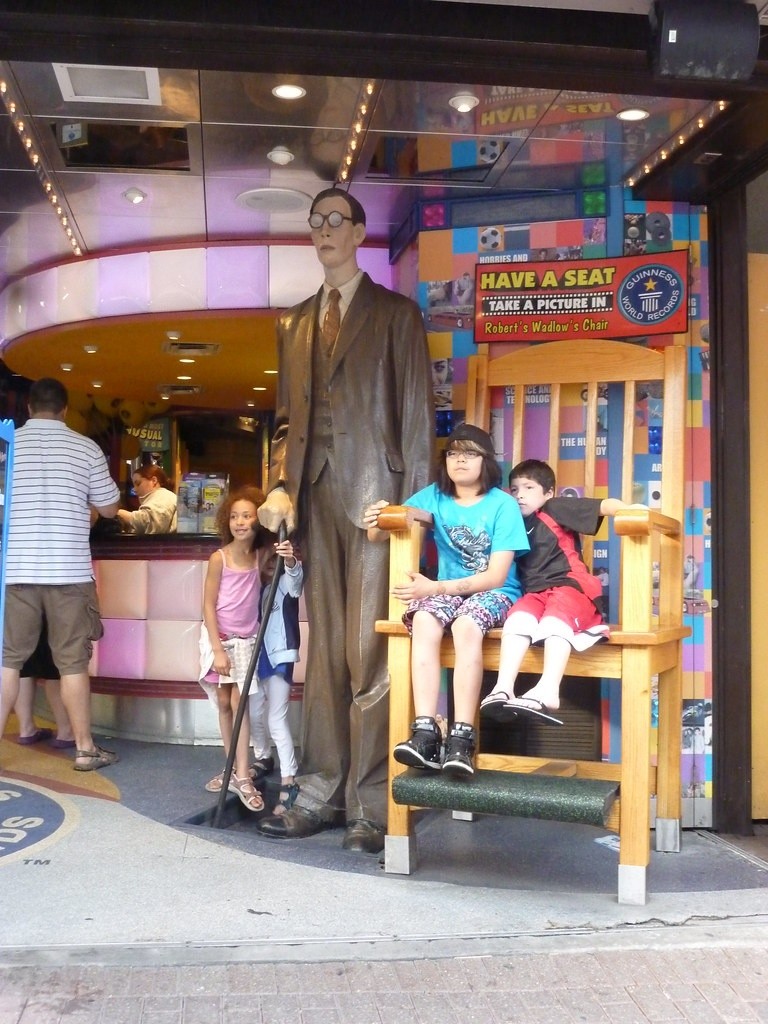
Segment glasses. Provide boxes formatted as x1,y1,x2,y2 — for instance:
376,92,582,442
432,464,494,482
446,450,478,458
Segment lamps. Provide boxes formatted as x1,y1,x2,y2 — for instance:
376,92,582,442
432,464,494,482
92,381,102,387
125,190,145,203
167,331,180,339
161,394,169,399
85,346,97,353
271,82,307,99
616,106,649,121
449,91,480,112
267,147,295,164
62,364,72,371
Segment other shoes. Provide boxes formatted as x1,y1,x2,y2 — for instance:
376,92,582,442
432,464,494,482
54,735,95,748
18,728,52,745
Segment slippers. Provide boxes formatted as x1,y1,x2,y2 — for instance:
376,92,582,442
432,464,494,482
503,695,563,725
479,691,516,723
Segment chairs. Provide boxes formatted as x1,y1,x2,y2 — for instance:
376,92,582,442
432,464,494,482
374,339,694,902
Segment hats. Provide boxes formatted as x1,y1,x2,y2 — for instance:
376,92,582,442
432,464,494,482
446,424,493,451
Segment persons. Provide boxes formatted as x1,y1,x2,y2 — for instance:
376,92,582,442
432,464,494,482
254,189,435,854
250,541,306,816
0,377,122,772
361,425,532,777
199,485,267,811
477,459,656,725
117,464,178,535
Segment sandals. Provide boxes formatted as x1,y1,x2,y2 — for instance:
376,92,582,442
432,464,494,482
247,757,275,780
272,782,300,816
73,747,119,771
205,767,236,793
228,774,265,812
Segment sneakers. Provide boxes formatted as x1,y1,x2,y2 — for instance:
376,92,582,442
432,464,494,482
393,716,445,770
442,721,477,774
